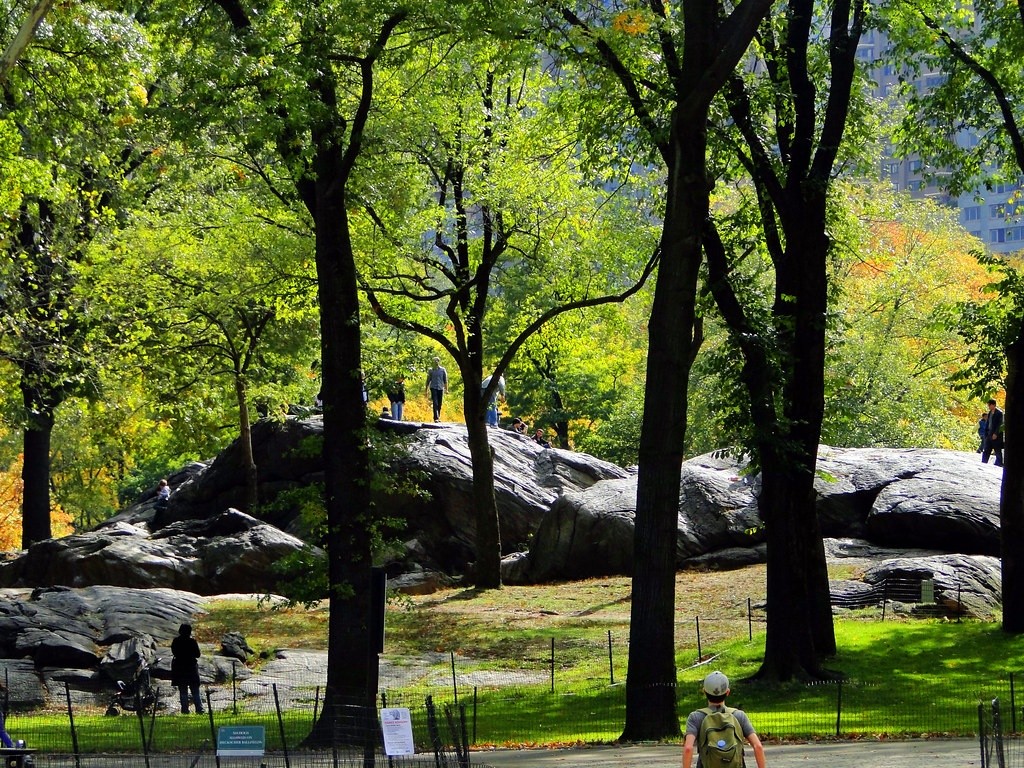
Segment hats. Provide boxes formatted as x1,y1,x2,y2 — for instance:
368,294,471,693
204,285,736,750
704,671,729,696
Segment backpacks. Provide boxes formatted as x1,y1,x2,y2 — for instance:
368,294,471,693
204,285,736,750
697,706,744,768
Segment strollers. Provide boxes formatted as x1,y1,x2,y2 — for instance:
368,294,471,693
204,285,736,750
105,657,167,718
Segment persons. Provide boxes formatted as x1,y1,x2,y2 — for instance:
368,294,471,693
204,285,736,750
391,379,406,420
361,372,368,407
507,417,554,449
481,373,505,428
0,684,14,748
425,358,448,422
682,672,768,768
171,624,206,715
972,413,988,453
152,478,170,523
982,400,1003,466
381,407,393,419
